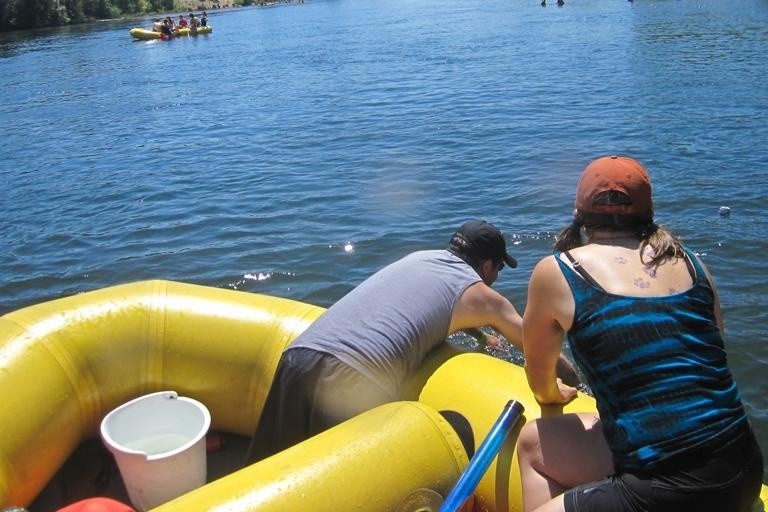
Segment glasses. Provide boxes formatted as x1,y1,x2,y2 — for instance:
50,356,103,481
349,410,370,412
494,257,504,270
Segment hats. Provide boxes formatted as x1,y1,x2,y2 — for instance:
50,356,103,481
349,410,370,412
576,157,652,214
450,221,517,268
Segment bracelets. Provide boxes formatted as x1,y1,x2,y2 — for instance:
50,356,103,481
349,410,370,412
478,331,485,344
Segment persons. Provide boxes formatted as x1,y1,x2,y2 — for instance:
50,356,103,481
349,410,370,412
238,219,577,468
187,13,200,29
515,155,762,512
176,15,187,29
199,11,208,27
153,9,176,38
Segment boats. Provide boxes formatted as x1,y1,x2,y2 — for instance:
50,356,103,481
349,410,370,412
0,280,768,512
129,25,212,38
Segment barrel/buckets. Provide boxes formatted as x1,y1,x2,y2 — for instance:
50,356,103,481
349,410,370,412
99,391,210,512
